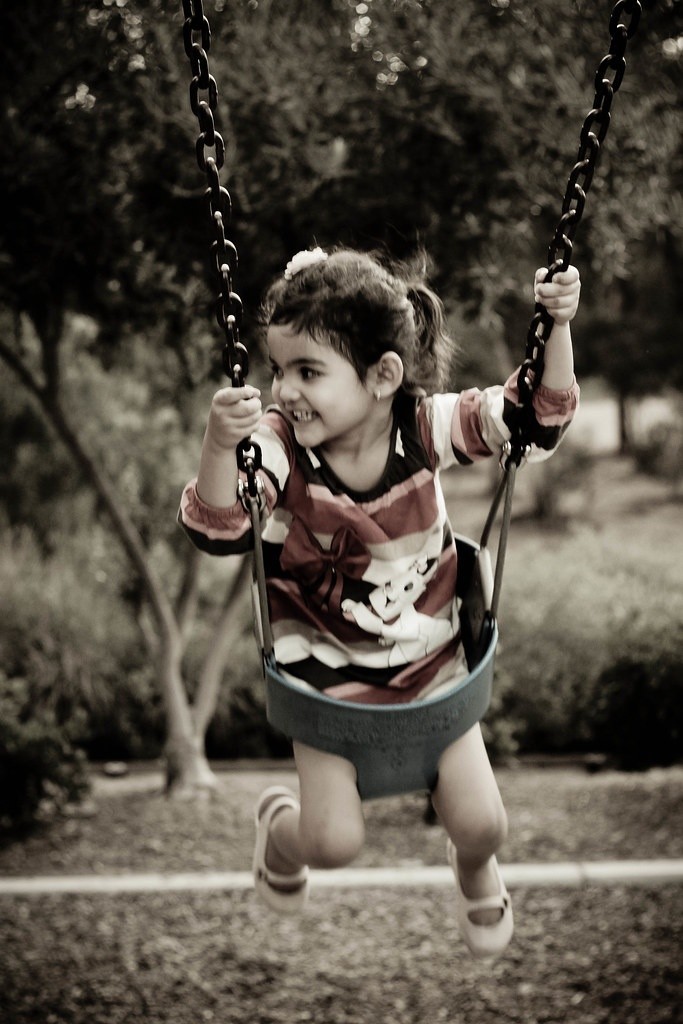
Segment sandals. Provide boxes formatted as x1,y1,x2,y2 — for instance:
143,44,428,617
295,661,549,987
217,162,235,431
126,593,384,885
444,833,513,956
253,785,313,918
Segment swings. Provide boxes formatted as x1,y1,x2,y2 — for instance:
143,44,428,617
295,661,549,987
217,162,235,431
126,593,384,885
180,0,646,800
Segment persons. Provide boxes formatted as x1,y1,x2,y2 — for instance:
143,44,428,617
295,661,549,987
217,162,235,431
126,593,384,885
175,242,583,958
